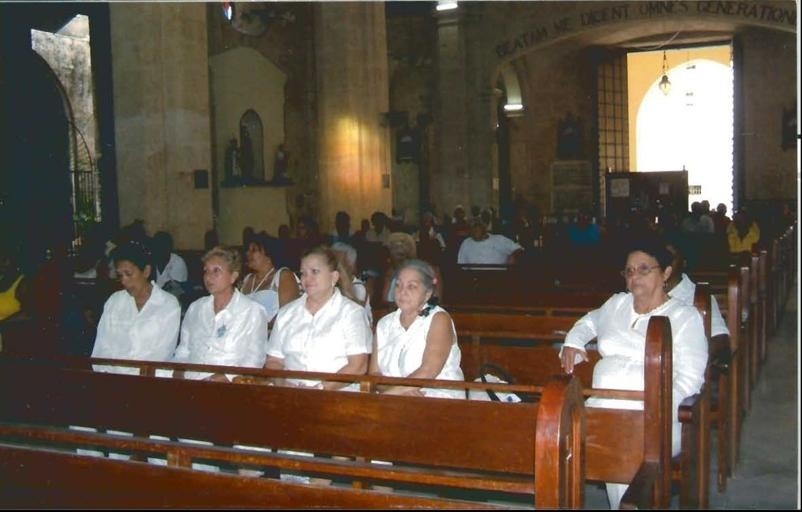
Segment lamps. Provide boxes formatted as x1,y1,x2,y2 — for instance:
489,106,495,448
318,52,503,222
659,50,673,97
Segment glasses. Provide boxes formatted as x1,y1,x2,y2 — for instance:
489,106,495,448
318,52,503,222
620,265,660,276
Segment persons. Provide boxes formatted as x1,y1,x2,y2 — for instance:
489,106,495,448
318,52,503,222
656,197,760,253
233,246,373,486
66,240,182,467
366,205,525,300
150,231,189,294
358,261,467,493
1,254,33,323
242,211,350,274
558,236,712,511
146,242,270,474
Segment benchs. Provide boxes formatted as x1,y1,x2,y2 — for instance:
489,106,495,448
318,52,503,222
0,223,797,511
